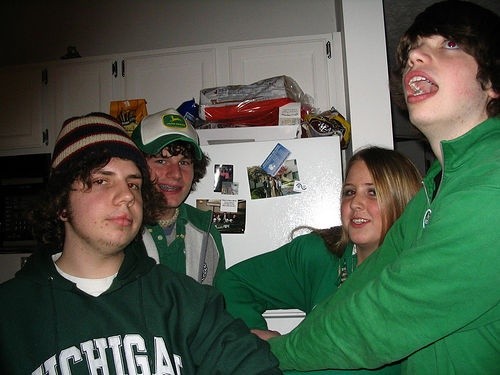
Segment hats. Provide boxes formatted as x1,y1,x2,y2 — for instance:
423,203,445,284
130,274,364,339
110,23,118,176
49,111,144,177
131,108,202,161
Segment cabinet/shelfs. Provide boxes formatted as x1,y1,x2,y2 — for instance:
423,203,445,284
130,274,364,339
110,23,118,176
1,31,348,158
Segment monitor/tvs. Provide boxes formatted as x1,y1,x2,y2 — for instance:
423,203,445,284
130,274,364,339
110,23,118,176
0,153,60,254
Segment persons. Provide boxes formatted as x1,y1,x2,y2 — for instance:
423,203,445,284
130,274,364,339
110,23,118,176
250,0,500,375
130,108,226,286
212,165,283,233
0,112,284,375
215,143,424,375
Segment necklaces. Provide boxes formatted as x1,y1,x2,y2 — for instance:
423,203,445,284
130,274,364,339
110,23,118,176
154,207,179,227
